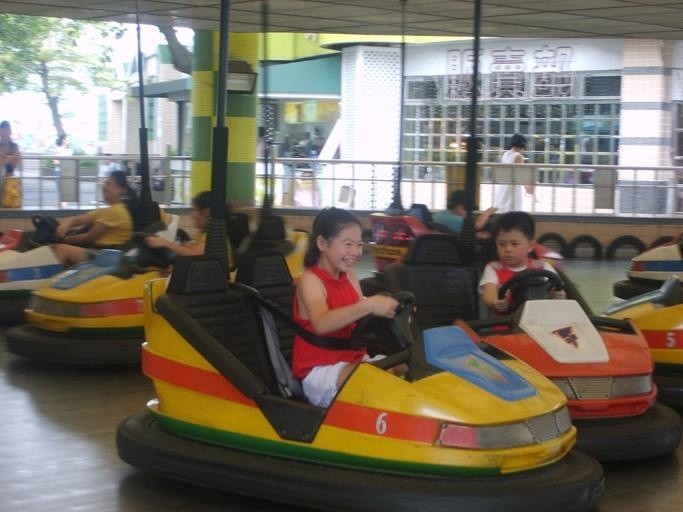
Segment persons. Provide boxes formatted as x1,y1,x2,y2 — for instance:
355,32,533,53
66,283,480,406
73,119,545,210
476,211,568,332
52,132,70,188
429,188,498,238
257,125,271,158
0,118,22,194
50,166,138,272
491,130,536,212
286,203,410,410
143,188,233,281
97,152,121,178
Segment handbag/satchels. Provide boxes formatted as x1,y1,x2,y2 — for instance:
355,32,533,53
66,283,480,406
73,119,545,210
3,175,22,209
